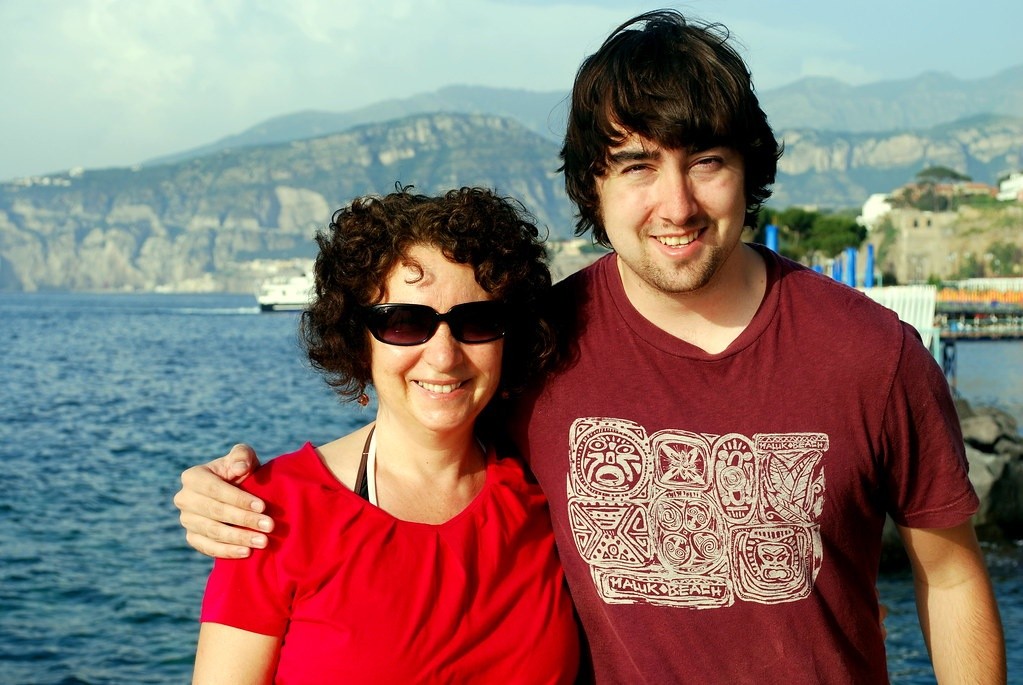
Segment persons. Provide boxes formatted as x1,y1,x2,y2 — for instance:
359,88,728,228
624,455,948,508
174,8,1007,685
191,180,581,685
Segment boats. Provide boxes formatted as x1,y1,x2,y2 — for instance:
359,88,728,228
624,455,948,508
254,253,317,311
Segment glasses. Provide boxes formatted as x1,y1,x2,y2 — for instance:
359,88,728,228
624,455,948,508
350,300,508,346
